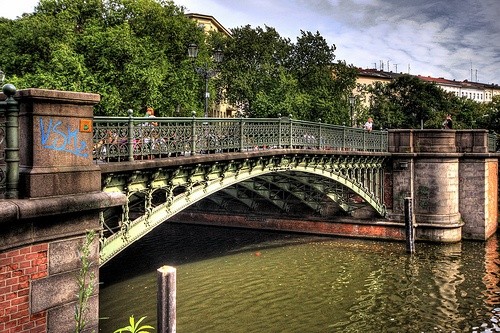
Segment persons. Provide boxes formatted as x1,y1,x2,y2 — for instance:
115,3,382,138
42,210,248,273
445,118,453,129
364,117,374,130
94,108,316,158
